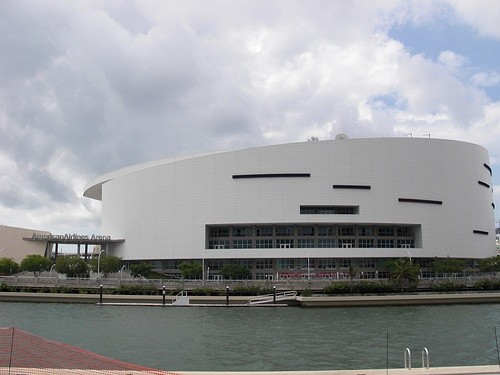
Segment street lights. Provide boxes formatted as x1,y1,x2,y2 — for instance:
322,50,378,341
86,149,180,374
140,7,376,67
121,264,127,281
304,251,311,281
49,264,56,278
97,249,106,279
